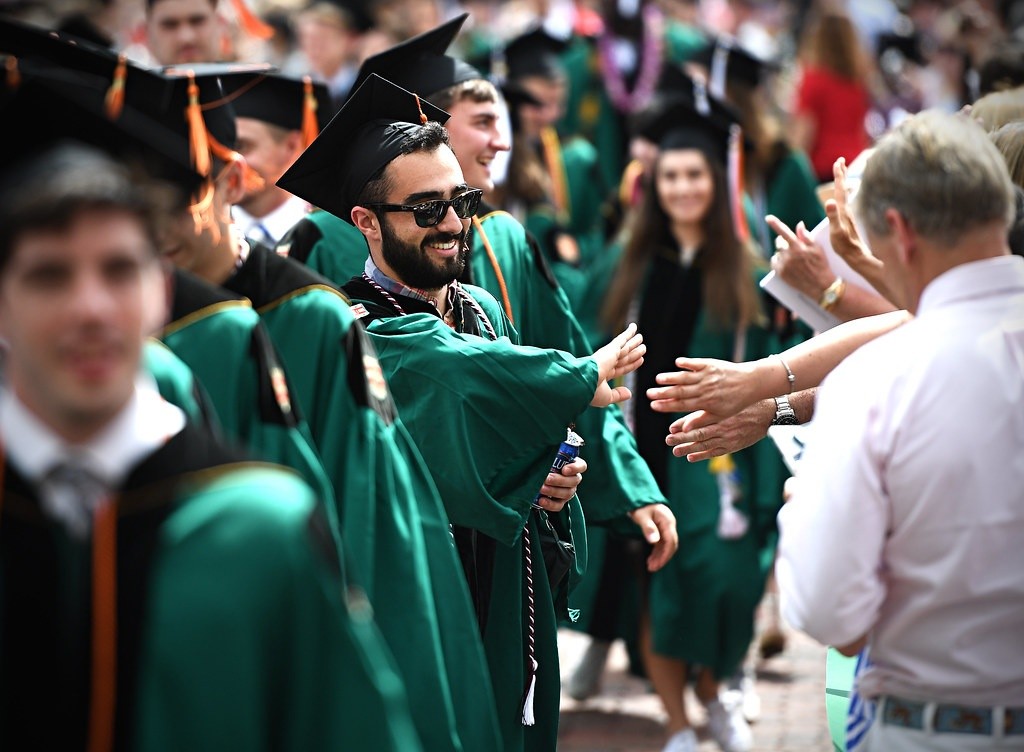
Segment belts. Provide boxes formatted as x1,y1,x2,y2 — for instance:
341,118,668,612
883,695,1024,735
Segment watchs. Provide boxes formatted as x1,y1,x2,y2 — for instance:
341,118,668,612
771,397,798,426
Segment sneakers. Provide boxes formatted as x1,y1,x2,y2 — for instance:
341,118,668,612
663,728,697,752
706,701,752,752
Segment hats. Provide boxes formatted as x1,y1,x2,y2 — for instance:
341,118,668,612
619,34,781,257
275,12,512,325
0,16,337,247
463,22,573,211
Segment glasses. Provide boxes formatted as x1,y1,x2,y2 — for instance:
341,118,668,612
363,187,483,228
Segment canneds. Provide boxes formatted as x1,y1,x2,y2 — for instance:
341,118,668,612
531,428,583,510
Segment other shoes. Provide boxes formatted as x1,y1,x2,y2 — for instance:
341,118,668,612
739,676,762,721
566,657,604,700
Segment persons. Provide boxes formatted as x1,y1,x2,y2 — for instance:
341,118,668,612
0,0,1024,752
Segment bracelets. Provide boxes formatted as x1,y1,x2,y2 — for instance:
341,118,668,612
774,353,796,393
818,275,846,313
